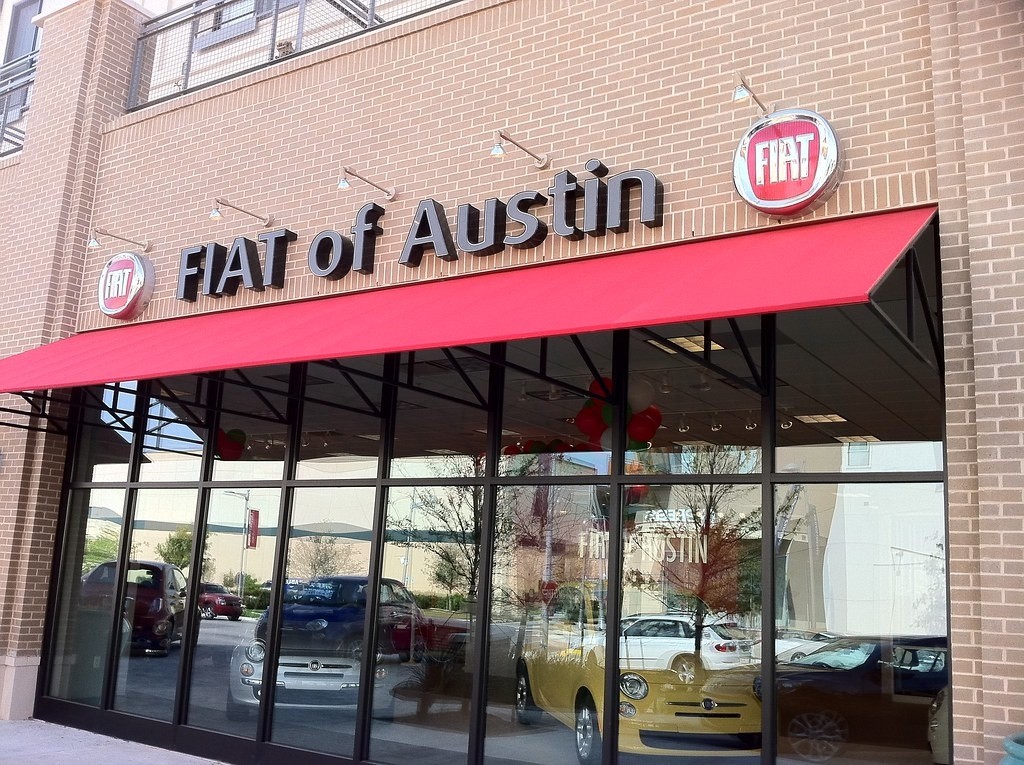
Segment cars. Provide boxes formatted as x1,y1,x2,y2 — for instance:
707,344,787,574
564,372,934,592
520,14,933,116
571,614,753,671
198,583,242,621
227,578,431,725
82,560,202,657
750,627,839,662
516,581,780,765
698,634,948,762
255,576,431,664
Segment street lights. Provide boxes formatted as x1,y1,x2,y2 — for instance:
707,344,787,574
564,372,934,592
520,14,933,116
223,490,250,598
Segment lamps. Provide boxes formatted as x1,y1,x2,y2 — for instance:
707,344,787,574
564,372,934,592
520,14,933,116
490,131,552,170
338,167,397,201
209,198,273,228
730,72,774,118
506,367,792,444
246,429,332,449
87,227,152,252
